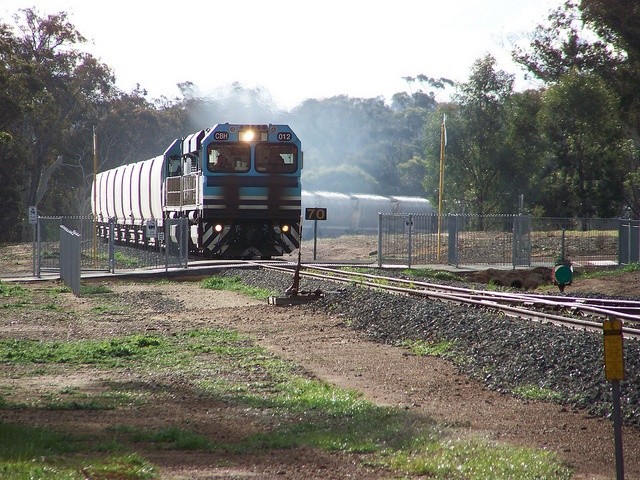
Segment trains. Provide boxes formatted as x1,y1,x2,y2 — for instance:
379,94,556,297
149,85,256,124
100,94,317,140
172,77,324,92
90,123,431,260
